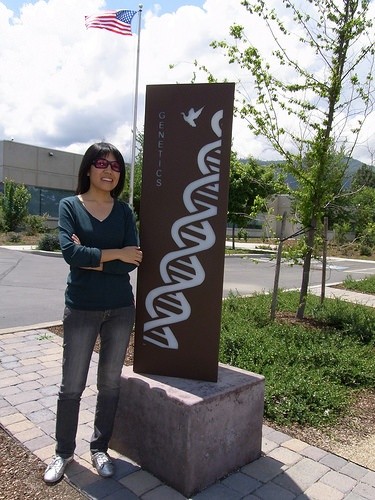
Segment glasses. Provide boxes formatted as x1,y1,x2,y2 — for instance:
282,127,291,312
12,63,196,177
92,158,123,172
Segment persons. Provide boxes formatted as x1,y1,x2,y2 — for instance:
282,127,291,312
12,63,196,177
42,142,142,482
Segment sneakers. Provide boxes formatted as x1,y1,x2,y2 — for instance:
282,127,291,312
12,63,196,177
91,452,116,477
44,455,74,482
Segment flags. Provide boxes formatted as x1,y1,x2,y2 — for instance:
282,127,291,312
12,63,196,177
84,10,137,36
